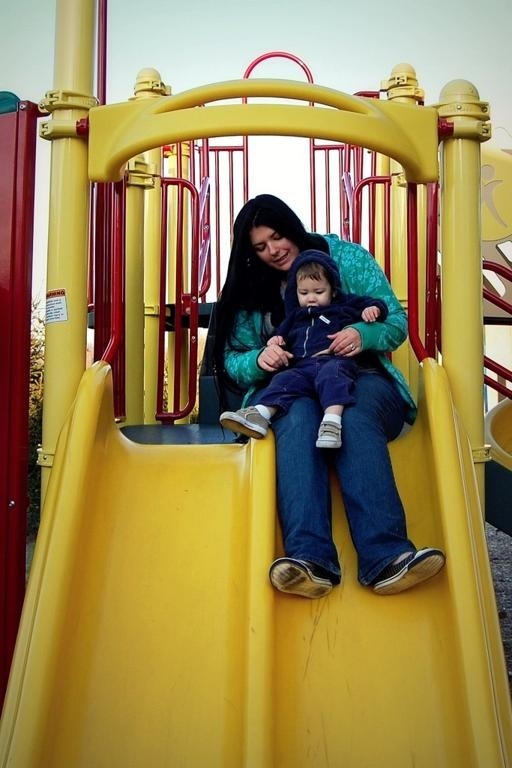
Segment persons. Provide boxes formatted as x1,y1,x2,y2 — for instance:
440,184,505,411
219,250,388,451
219,194,446,598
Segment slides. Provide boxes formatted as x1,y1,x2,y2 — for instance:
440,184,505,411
3,358,510,767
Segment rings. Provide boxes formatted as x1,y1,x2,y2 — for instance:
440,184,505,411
350,343,356,351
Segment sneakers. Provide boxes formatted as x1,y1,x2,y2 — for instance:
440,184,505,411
219,406,269,439
373,547,446,595
269,558,333,599
315,421,343,448
